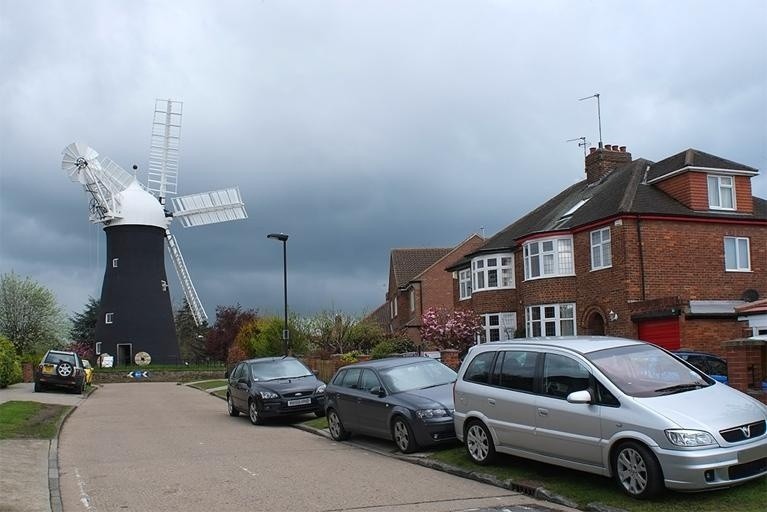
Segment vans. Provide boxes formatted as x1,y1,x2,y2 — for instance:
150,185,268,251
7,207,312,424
453,334,767,499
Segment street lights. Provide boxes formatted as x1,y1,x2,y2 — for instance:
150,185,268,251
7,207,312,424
266,232,290,357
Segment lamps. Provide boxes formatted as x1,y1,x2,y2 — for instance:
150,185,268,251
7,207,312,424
608,310,618,322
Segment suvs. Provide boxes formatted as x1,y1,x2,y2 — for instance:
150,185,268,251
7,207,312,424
33,349,86,395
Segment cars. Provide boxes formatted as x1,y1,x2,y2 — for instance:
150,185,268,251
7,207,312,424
634,351,767,391
321,353,462,454
80,360,94,385
225,354,326,425
